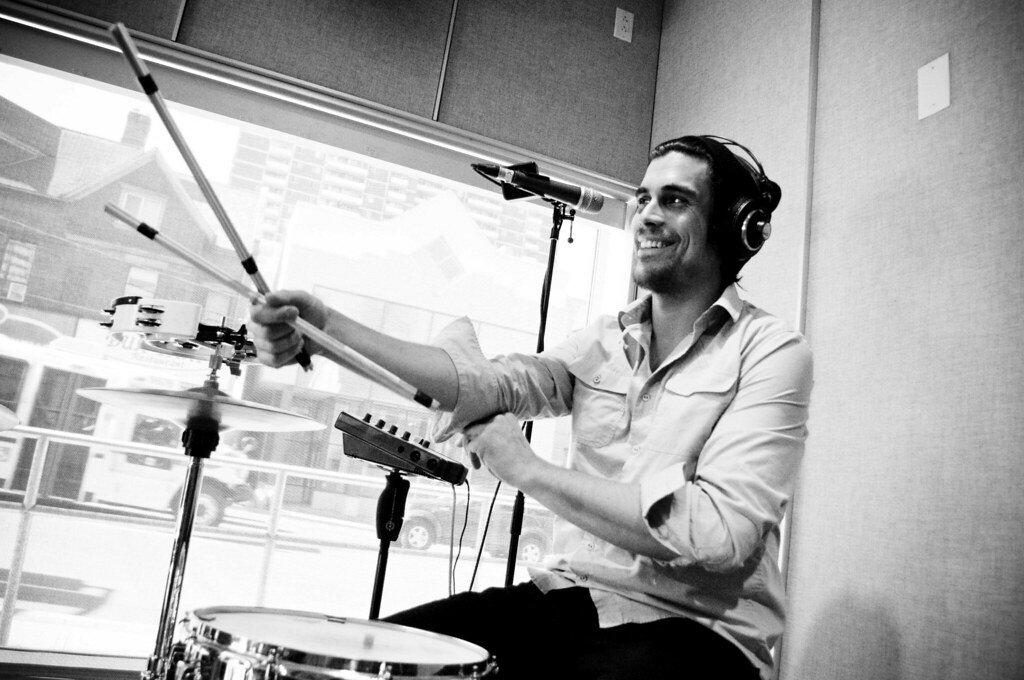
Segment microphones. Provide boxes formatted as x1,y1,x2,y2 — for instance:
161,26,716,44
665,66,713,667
476,163,605,213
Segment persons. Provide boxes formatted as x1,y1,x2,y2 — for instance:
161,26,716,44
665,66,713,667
247,135,814,680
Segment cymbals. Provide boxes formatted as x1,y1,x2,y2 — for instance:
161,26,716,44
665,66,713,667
75,384,329,435
99,320,115,328
134,317,161,329
103,307,116,316
137,303,165,313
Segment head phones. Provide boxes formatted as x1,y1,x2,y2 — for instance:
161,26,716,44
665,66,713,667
682,134,771,260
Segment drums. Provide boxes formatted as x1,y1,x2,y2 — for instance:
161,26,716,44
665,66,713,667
171,605,494,680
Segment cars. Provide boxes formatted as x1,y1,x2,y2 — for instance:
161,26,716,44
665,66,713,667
110,411,253,534
400,493,560,569
